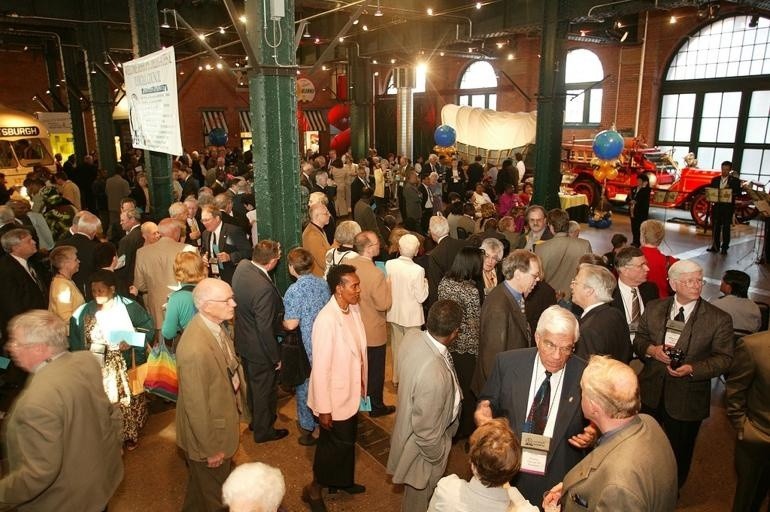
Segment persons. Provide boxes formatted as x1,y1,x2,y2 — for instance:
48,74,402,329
192,144,253,179
429,172,442,213
134,218,201,341
444,192,464,217
344,231,395,417
633,260,736,493
448,201,476,244
474,305,601,512
114,208,145,291
302,203,335,278
467,155,484,185
601,235,630,266
479,238,504,292
69,270,155,450
0,228,49,390
421,153,440,180
468,218,507,252
377,214,396,242
140,222,161,247
403,171,423,231
55,153,63,172
311,171,341,230
496,159,514,195
162,252,232,349
470,249,543,396
387,152,395,168
427,417,539,512
497,184,525,233
623,174,652,247
173,169,182,202
92,167,109,228
519,182,534,208
566,220,580,239
223,461,286,512
570,263,632,366
179,168,200,201
475,202,499,237
725,324,770,512
176,277,251,512
300,148,326,169
482,158,498,190
327,149,338,169
210,170,228,197
86,242,138,300
397,157,410,226
168,202,189,247
0,309,123,512
173,152,192,168
613,248,659,359
105,165,132,224
323,220,362,289
201,205,250,286
118,143,145,176
359,158,371,182
61,214,102,297
463,191,482,222
354,187,386,261
390,155,401,210
328,158,348,217
385,234,429,388
445,158,465,200
40,186,74,239
63,154,77,182
242,193,258,249
639,219,683,301
282,247,332,446
232,180,255,223
0,204,22,236
302,192,335,245
388,218,425,260
23,164,52,197
54,170,82,211
226,178,242,200
474,184,492,216
499,216,520,252
418,174,434,230
424,216,474,308
303,264,368,512
536,208,594,295
386,300,464,512
439,247,485,441
438,156,449,182
515,153,525,186
212,194,238,226
351,166,373,219
710,270,761,337
374,159,390,221
707,161,743,256
48,245,86,323
0,173,16,206
413,154,424,176
345,156,358,183
126,167,151,215
73,156,96,215
182,195,205,248
6,197,56,251
519,205,552,258
542,355,679,512
232,239,289,443
300,163,314,193
107,198,136,244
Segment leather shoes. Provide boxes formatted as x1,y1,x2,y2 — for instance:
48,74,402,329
295,419,313,437
254,428,289,443
298,432,320,447
247,414,279,432
721,249,728,257
705,247,721,253
369,405,397,418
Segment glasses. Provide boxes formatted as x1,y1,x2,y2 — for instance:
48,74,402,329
666,278,706,287
367,240,379,247
198,215,218,224
479,249,486,256
539,342,575,353
450,157,458,162
570,277,579,286
624,261,649,268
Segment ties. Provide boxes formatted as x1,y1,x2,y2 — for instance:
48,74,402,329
673,305,688,322
213,231,218,266
427,187,433,204
518,297,530,340
221,166,223,172
330,159,332,164
432,166,435,172
524,369,552,435
628,285,645,334
25,262,47,301
362,178,369,188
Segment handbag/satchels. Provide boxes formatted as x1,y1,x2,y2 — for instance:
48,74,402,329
142,332,179,404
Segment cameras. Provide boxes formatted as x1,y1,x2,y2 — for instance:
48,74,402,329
666,348,684,370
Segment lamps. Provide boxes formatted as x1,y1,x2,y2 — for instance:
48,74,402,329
162,23,170,29
375,10,382,14
495,42,505,48
240,15,246,26
304,32,310,36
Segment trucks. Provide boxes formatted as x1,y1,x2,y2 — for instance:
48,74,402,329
562,133,721,229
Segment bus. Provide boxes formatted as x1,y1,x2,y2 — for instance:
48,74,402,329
0,111,62,190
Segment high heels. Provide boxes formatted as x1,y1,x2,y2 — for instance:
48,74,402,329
300,485,328,511
328,484,367,495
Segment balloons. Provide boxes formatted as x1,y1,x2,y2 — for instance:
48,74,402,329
591,159,618,182
433,146,455,159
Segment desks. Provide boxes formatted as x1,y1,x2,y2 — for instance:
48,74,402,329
560,186,590,223
